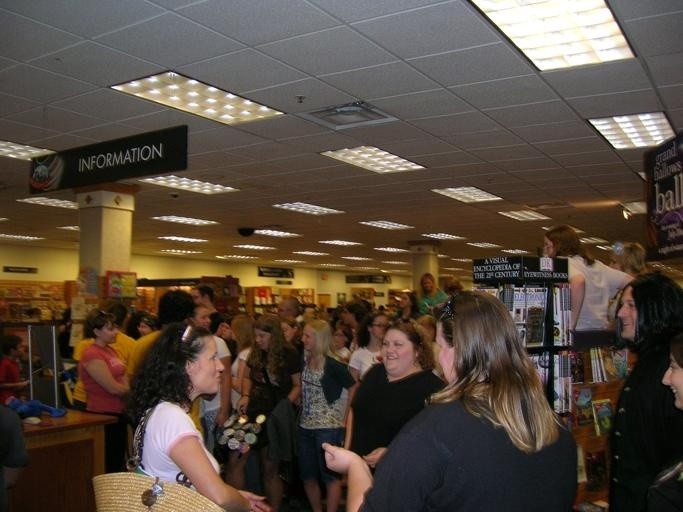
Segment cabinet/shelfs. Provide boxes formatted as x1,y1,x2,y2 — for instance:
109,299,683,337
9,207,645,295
197,276,315,319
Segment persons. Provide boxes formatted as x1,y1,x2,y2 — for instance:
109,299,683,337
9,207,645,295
0,224,682,512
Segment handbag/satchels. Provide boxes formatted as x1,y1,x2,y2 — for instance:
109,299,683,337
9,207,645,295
90,407,226,512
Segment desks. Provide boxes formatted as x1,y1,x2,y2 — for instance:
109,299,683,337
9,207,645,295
6,408,119,512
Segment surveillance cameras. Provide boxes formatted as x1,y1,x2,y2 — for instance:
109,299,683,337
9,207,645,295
237,227,255,236
